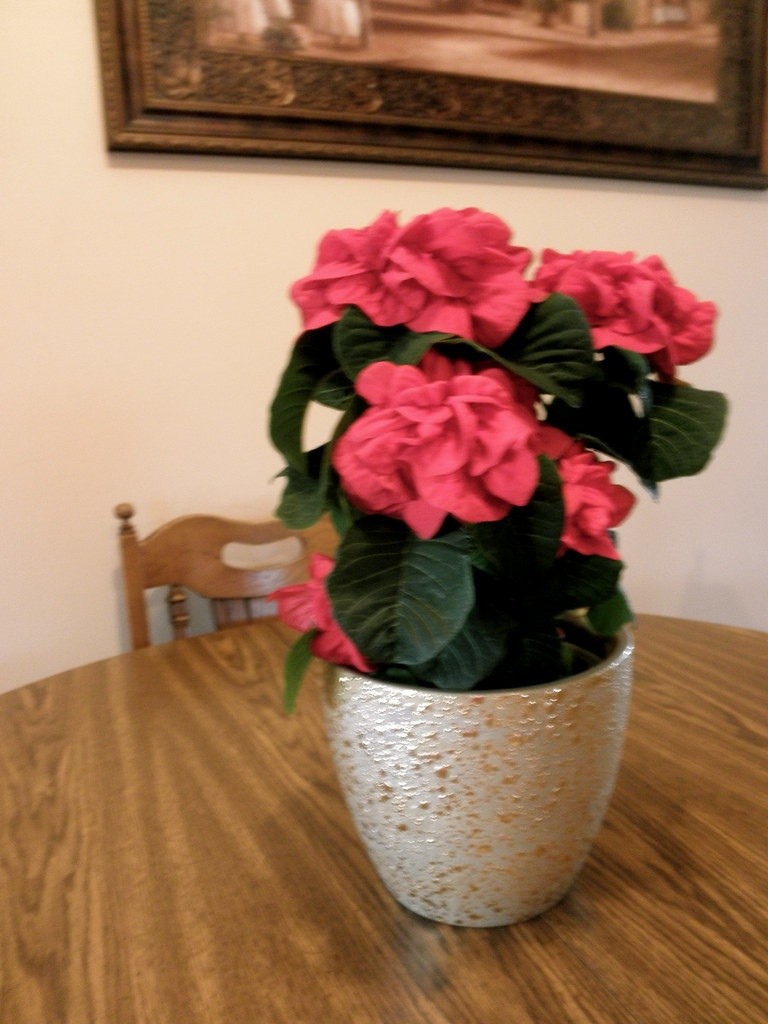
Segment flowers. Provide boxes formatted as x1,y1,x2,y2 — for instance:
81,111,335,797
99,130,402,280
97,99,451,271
267,203,728,717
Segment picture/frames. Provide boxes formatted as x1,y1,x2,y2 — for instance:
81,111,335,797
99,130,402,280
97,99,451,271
92,0,768,193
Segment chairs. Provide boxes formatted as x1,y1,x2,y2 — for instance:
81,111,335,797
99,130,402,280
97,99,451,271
115,501,341,650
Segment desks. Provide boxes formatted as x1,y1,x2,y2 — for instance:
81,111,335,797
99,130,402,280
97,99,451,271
0,611,768,1024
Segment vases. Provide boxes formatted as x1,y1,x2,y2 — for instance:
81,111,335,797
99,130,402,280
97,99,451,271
324,622,633,930
